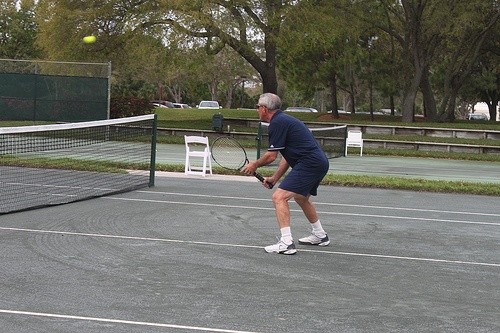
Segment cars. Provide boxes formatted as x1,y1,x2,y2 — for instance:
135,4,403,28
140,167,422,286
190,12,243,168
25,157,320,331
327,109,425,118
149,103,168,108
195,101,222,109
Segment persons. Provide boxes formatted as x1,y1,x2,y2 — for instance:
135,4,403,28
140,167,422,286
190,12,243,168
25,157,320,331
240,93,331,254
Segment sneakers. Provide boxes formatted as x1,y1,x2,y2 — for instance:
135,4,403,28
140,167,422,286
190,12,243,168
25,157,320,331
264,238,298,255
298,232,330,246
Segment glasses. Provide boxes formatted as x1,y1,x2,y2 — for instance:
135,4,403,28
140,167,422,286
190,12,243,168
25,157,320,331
256,104,266,110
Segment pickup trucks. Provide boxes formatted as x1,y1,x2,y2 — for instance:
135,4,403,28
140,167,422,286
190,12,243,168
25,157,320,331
469,112,488,121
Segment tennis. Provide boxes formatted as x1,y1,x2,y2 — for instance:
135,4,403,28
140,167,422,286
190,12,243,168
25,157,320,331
83,36,96,44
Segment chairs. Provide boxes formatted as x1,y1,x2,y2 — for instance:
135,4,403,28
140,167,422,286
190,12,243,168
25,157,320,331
183,134,213,178
345,129,363,157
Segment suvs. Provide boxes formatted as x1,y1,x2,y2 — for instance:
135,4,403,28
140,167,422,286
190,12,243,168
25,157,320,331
150,100,175,108
173,103,192,109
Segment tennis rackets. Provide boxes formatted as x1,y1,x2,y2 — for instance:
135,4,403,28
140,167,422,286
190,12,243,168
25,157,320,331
210,136,273,189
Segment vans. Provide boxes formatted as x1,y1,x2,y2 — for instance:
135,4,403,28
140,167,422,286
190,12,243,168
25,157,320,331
282,107,318,112
